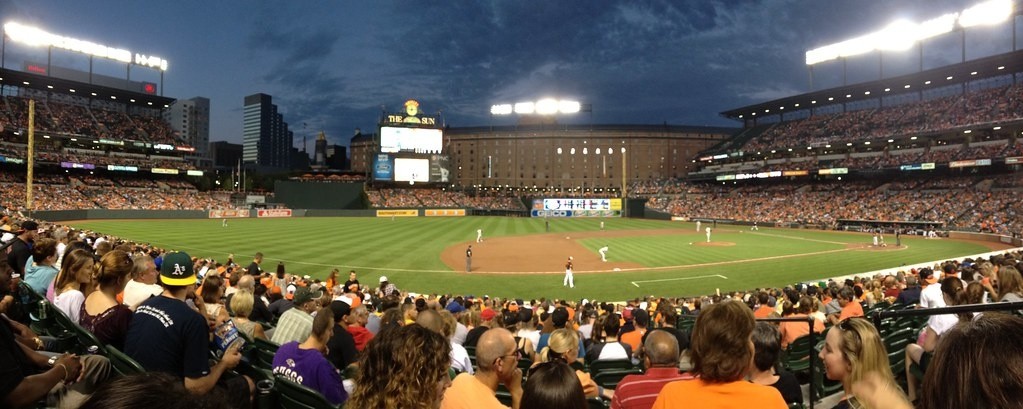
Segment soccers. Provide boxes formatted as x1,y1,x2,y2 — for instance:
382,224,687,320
689,242,693,245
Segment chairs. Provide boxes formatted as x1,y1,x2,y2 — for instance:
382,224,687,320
0,243,1023,409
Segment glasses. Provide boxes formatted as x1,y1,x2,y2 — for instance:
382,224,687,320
492,350,518,366
838,316,865,331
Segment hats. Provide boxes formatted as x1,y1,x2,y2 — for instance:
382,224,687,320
919,268,937,284
622,309,633,319
519,309,532,322
943,264,957,275
159,250,196,286
379,276,388,282
446,302,465,313
481,309,498,321
581,299,588,306
293,286,322,301
22,221,38,230
331,295,360,313
285,285,296,300
631,309,647,327
552,307,569,323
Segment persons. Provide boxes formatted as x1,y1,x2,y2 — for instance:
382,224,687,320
705,226,711,243
0,82,1023,409
465,245,472,272
563,256,575,289
696,221,701,232
599,246,608,262
751,221,758,231
477,229,483,243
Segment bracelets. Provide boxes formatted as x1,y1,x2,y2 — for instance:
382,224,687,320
57,363,68,383
47,356,59,368
